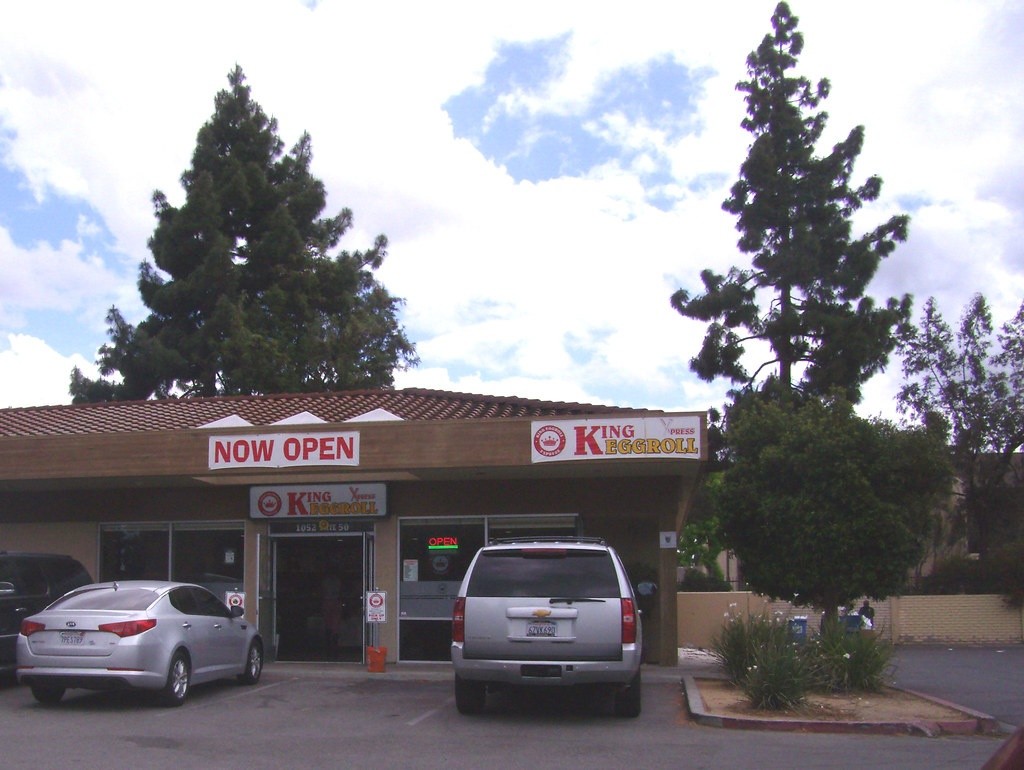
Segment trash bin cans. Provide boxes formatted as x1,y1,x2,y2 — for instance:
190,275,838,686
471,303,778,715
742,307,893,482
820,613,864,644
789,617,808,645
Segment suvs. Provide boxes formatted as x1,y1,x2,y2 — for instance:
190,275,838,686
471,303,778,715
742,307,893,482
448,534,643,717
0,551,94,675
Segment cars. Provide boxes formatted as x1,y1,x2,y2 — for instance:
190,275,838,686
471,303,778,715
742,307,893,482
16,582,264,708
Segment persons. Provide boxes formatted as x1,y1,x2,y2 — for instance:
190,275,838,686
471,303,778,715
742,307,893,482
859,601,875,630
